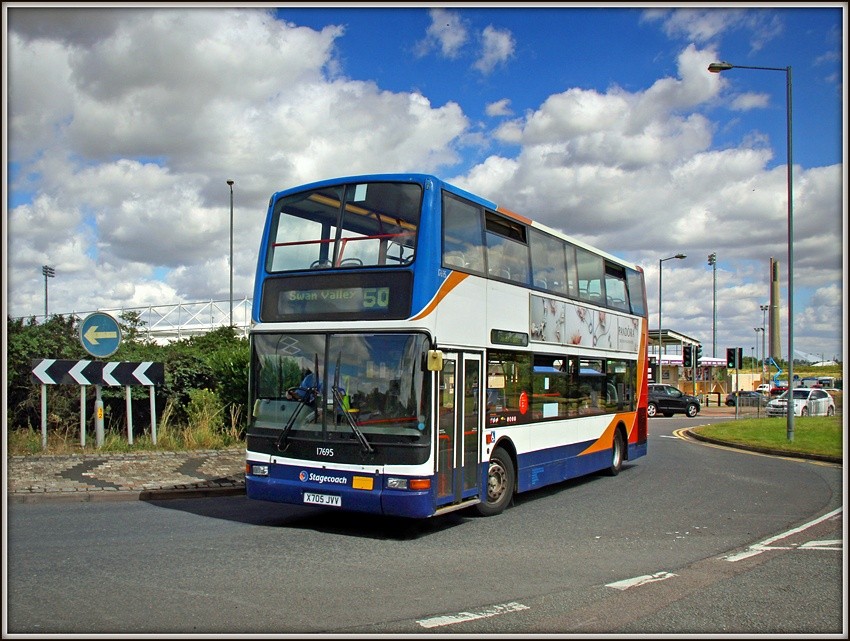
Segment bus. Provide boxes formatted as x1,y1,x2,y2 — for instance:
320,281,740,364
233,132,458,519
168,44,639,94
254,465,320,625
241,172,650,523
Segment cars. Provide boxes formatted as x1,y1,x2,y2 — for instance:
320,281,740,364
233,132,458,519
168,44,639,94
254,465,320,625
756,383,772,393
770,386,789,395
764,387,836,418
725,390,775,408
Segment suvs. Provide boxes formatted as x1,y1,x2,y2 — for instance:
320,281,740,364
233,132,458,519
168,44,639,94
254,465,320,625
647,383,701,418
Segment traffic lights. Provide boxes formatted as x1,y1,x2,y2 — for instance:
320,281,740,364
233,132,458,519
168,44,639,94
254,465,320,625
693,345,703,368
683,346,692,368
726,347,735,368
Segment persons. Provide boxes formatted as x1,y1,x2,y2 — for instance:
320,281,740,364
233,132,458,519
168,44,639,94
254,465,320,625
286,355,348,416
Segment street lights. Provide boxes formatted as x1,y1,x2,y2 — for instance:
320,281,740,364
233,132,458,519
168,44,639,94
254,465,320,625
753,327,762,374
658,253,688,386
707,251,718,357
42,264,56,325
760,304,770,404
226,177,235,328
708,61,795,442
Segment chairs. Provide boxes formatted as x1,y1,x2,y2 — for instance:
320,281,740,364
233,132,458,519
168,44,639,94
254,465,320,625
308,250,634,317
